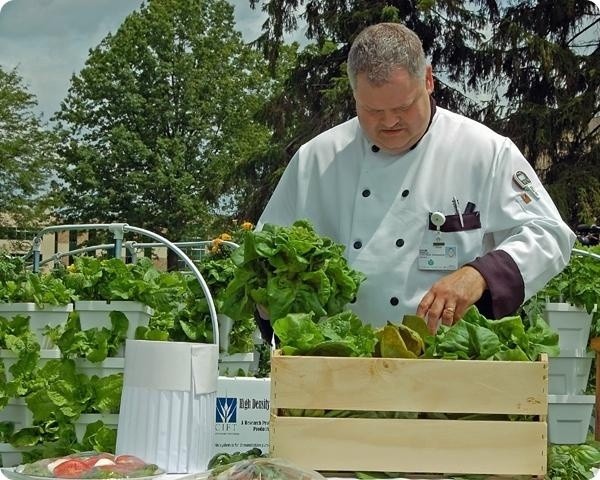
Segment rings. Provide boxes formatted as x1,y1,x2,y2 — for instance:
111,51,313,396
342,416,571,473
443,308,455,313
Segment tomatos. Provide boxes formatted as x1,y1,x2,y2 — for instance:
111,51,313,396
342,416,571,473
51,453,145,476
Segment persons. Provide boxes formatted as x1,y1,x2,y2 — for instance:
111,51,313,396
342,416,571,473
252,23,577,346
445,248,455,257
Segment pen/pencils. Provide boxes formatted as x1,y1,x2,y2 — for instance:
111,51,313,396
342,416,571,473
453,195,465,228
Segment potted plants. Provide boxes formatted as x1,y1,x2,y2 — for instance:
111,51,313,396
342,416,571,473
1,217,600,470
